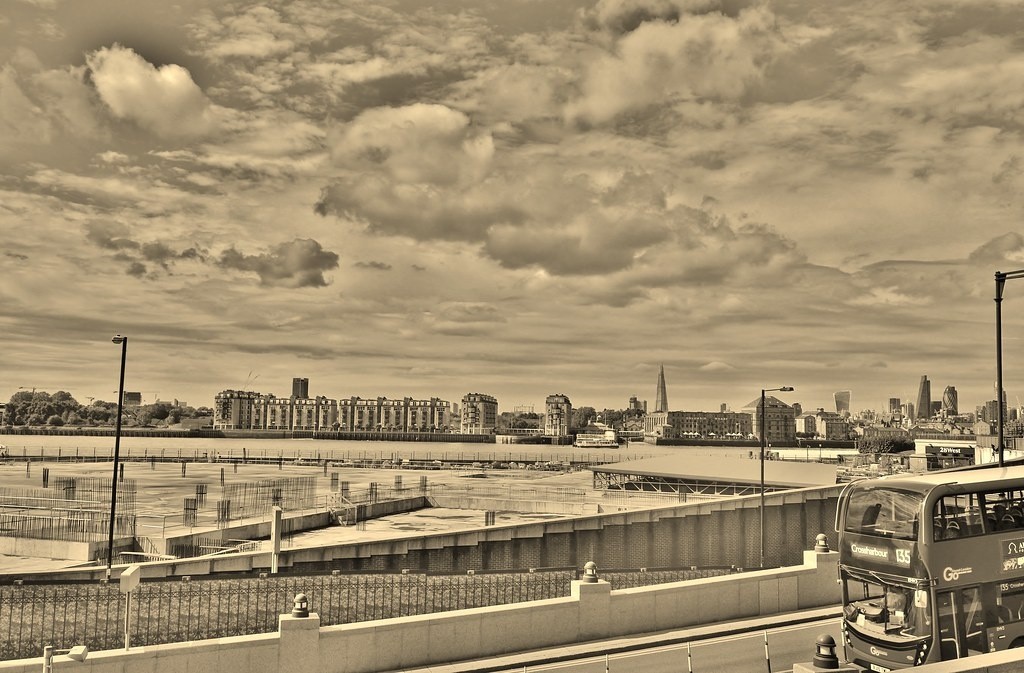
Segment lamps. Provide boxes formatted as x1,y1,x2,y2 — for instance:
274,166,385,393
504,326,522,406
292,594,309,617
814,533,830,552
583,561,599,583
813,635,838,669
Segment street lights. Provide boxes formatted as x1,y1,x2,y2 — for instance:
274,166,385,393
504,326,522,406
103,334,128,586
759,386,795,570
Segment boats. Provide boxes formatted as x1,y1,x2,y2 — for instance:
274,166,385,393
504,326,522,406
574,430,620,448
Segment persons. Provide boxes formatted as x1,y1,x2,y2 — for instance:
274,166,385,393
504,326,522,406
863,585,906,615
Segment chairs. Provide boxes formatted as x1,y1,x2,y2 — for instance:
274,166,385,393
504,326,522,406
998,605,1012,623
933,503,1024,540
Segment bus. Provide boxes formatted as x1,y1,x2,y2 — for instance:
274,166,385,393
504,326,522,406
834,464,1024,673
